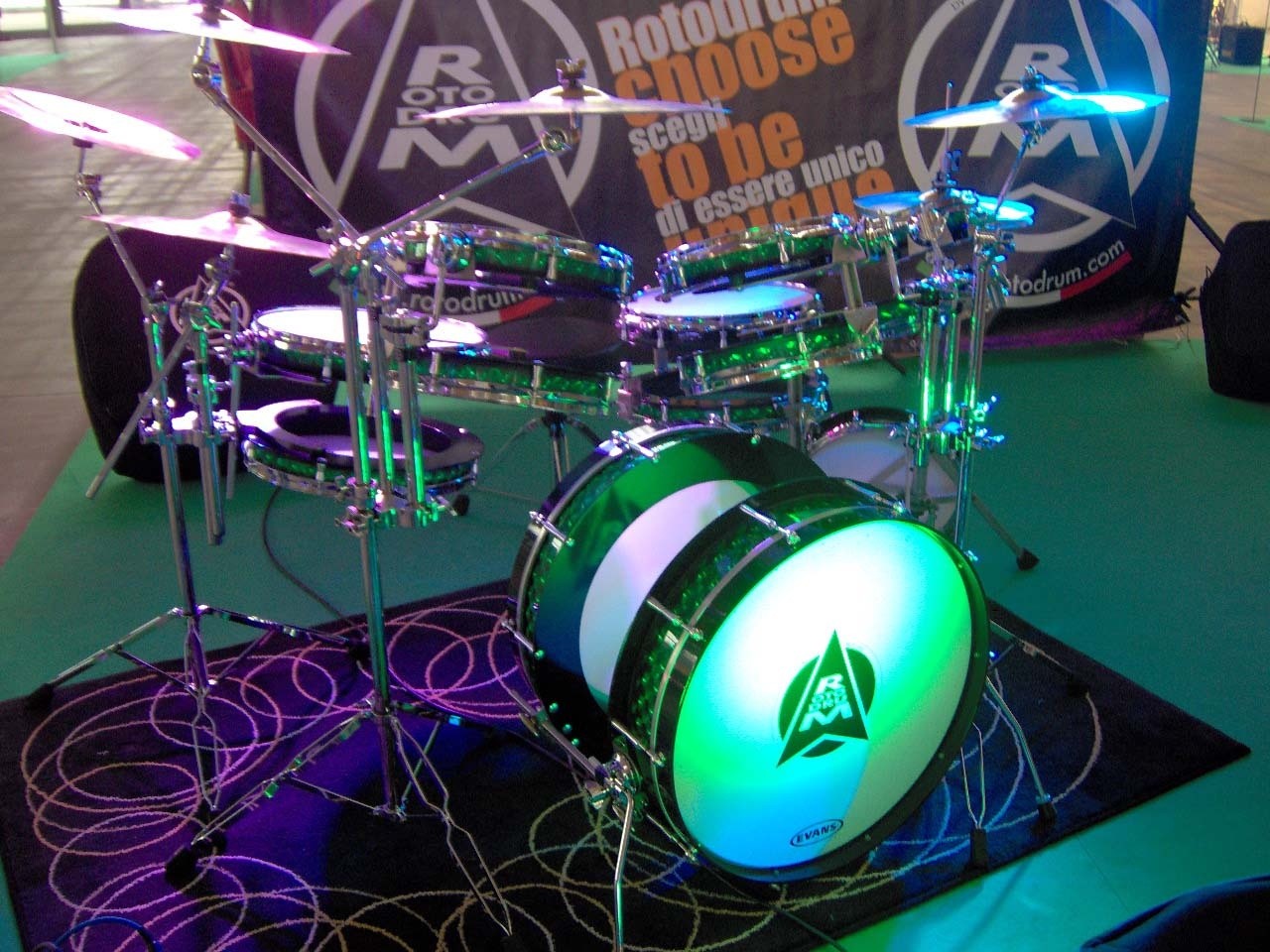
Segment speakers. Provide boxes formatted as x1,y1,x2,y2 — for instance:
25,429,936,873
1220,25,1265,65
1200,218,1270,400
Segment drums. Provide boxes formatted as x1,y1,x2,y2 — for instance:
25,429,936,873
608,280,827,345
503,415,993,890
673,303,881,391
653,212,841,298
805,403,971,534
869,269,1004,346
247,301,489,378
234,404,486,500
627,366,832,434
378,338,622,424
381,220,631,299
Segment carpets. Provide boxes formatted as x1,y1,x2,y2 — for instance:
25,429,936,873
2,559,1250,952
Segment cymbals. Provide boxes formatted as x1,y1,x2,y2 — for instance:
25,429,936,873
412,81,737,115
55,1,351,60
902,90,1170,128
76,205,341,261
0,82,199,167
852,187,1036,235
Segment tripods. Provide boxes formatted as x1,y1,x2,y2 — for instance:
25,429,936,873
876,425,1045,579
20,320,372,847
161,512,547,878
450,408,615,514
